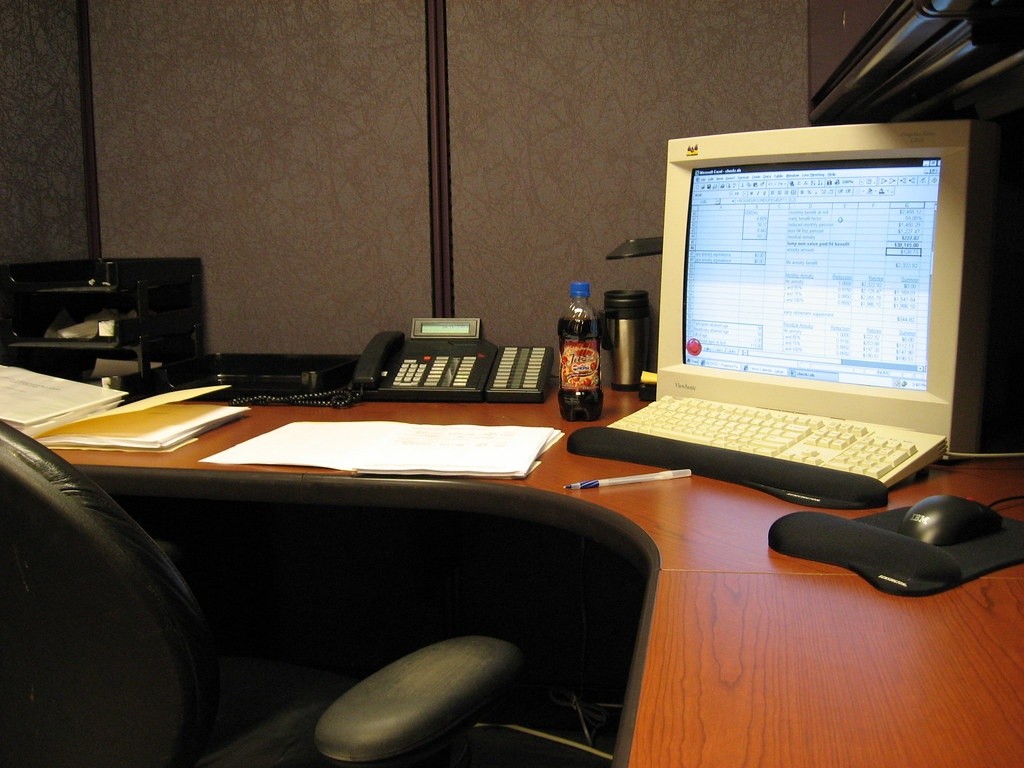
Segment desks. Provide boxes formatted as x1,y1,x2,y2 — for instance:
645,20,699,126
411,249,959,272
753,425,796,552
50,384,1024,768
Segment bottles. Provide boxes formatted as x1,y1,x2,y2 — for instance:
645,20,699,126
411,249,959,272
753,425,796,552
557,281,603,421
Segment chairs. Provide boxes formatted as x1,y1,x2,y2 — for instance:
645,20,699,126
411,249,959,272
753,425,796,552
0,420,527,768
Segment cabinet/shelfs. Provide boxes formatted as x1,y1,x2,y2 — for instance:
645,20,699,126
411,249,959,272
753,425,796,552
0,258,203,395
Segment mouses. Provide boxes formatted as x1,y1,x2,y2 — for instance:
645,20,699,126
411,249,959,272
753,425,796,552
900,495,1002,547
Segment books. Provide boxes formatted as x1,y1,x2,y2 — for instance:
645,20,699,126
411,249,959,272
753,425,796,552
0,364,129,439
32,384,252,453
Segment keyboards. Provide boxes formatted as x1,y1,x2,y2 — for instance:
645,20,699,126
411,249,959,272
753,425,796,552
607,395,947,489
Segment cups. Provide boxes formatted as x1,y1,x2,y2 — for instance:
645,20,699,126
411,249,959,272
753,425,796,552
603,291,650,392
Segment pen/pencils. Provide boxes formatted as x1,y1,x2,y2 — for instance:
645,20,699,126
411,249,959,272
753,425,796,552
563,468,691,490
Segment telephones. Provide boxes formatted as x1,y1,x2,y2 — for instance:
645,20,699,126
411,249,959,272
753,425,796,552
343,317,555,403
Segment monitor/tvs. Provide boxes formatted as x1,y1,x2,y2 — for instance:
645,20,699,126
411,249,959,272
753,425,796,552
657,116,1024,461
